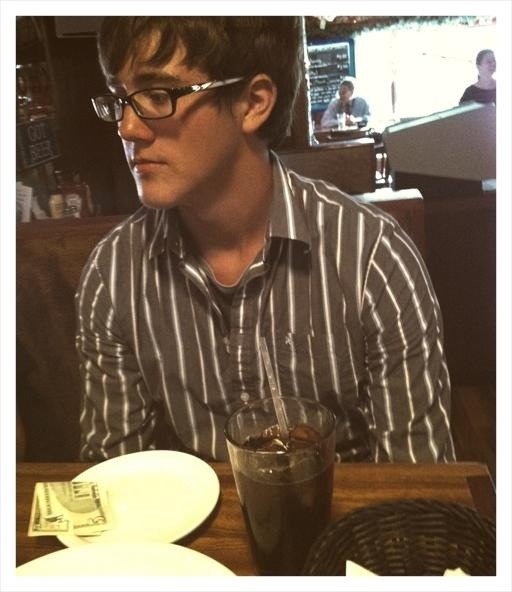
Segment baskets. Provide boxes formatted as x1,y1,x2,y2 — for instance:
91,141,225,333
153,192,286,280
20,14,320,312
299,496,496,575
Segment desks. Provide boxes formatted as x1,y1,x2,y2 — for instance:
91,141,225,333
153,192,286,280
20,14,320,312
15,457,496,575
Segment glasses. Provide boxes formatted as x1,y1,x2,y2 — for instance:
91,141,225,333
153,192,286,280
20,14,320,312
90,74,251,124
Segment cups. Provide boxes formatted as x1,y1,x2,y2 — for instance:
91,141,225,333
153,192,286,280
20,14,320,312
50,193,81,219
225,396,337,575
336,112,346,129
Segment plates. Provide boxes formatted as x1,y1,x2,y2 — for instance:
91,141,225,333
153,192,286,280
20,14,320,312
16,543,238,576
56,449,221,547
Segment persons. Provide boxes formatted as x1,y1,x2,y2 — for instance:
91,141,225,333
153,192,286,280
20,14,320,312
320,81,371,128
72,16,457,464
459,48,496,103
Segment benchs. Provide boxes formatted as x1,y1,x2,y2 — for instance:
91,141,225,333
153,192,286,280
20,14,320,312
353,189,429,263
276,137,376,195
17,215,126,455
